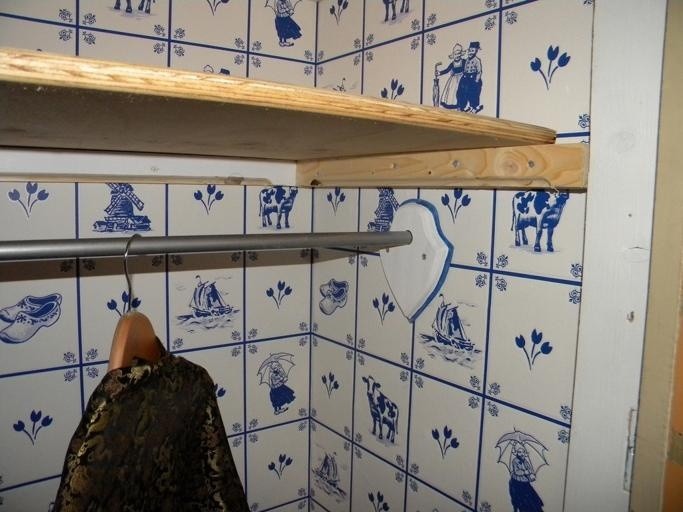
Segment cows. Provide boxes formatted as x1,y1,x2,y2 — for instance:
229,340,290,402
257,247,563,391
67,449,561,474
510,190,569,252
258,185,297,229
360,376,399,444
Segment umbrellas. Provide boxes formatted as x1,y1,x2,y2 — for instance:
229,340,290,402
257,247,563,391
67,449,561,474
433,62,442,107
495,428,549,480
265,0,302,14
257,352,296,387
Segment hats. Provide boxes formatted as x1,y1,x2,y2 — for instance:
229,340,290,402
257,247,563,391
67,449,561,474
469,42,480,48
449,43,466,58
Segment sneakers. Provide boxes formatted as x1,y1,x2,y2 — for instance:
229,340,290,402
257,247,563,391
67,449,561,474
319,279,348,315
0,294,63,344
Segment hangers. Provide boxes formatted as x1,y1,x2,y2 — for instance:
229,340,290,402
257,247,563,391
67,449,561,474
106,233,169,372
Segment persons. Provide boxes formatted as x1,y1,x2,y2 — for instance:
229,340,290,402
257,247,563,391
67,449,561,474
509,443,544,512
435,44,466,109
275,0,302,46
269,362,296,415
456,42,484,114
